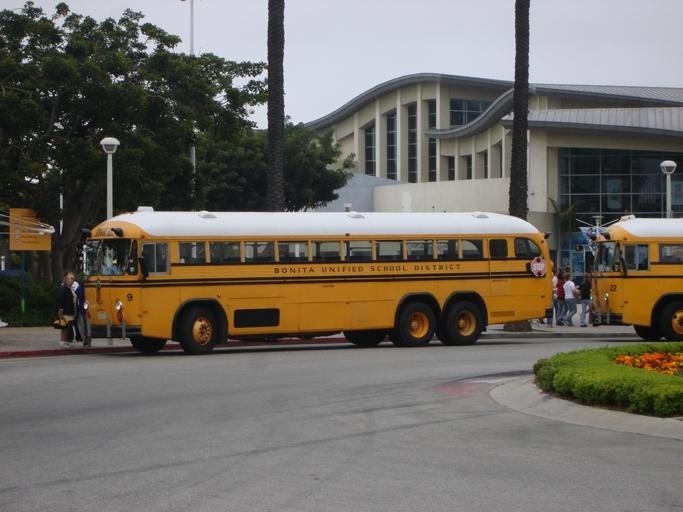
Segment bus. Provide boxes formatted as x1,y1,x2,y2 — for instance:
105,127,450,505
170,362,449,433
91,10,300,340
80,206,554,355
586,215,683,341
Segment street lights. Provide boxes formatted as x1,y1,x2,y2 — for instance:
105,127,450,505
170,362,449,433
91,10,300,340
660,159,678,218
100,137,121,219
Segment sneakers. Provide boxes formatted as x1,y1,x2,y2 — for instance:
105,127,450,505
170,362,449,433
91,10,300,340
59,335,91,348
536,318,600,327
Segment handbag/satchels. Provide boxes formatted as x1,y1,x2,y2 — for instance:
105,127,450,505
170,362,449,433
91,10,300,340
53,310,68,330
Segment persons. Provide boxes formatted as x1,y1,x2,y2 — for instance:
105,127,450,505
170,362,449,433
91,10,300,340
551,268,563,326
561,273,582,326
52,270,91,349
577,273,594,327
556,271,568,326
